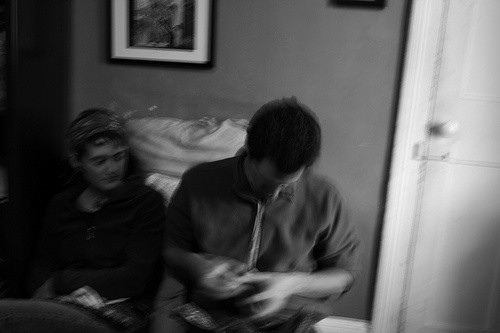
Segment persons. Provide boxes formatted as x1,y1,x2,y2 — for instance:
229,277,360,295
0,107,171,333
164,95,367,333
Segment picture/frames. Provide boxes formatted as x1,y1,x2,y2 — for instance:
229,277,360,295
107,0,214,71
